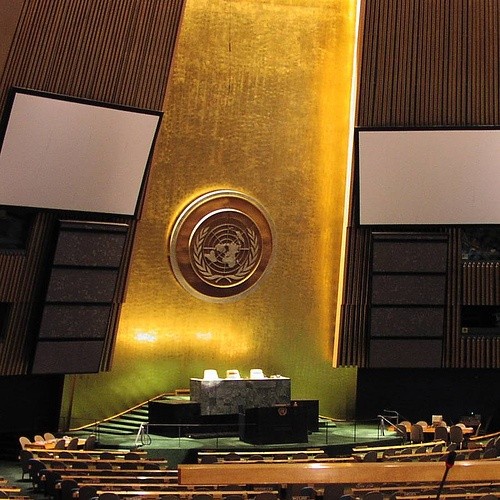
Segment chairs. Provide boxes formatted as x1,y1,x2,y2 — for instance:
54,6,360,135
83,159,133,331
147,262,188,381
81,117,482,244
17,415,500,500
226,369,241,379
204,370,218,378
249,369,264,379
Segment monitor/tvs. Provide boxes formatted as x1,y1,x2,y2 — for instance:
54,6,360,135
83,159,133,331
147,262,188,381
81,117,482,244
353,124,499,228
1,86,164,219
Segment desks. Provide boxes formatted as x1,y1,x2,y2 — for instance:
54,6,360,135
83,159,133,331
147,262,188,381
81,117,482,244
190,376,292,415
